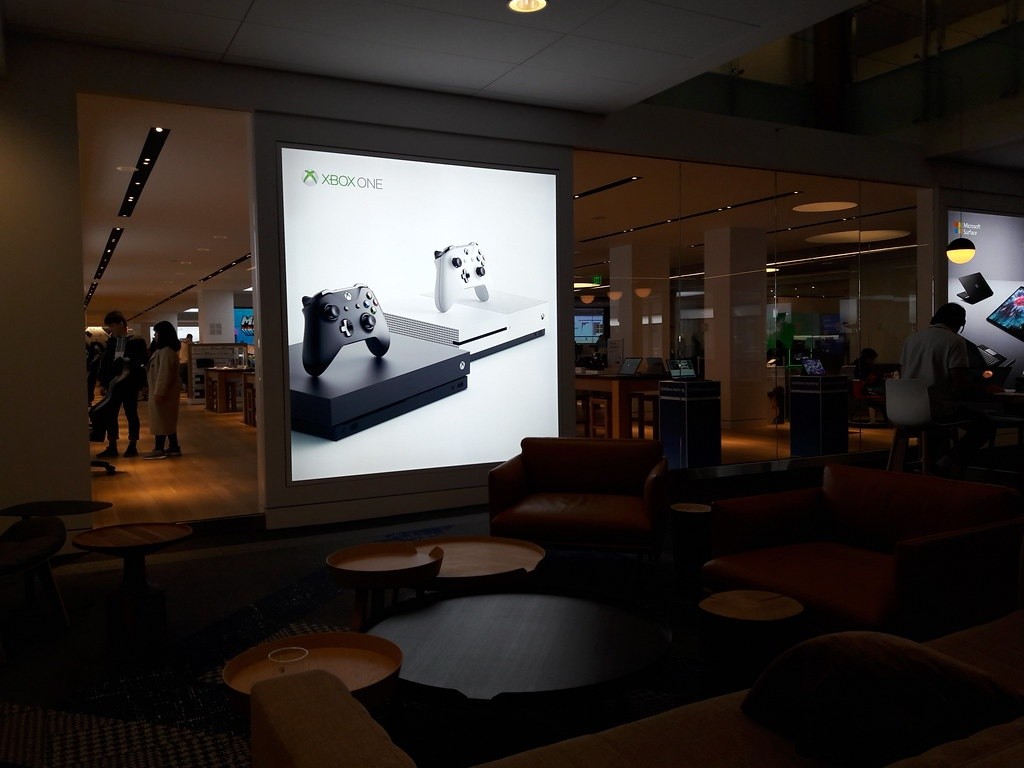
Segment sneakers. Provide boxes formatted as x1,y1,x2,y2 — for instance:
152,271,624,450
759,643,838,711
165,446,181,456
144,450,167,459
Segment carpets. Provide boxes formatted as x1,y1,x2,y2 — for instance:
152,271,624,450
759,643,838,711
55,524,761,767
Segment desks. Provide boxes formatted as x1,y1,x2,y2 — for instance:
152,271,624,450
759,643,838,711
573,374,668,440
0,500,114,520
984,391,1024,404
242,372,258,427
670,503,710,512
71,520,193,660
223,628,404,702
400,536,546,609
204,367,255,413
363,593,662,700
322,541,444,631
694,591,805,623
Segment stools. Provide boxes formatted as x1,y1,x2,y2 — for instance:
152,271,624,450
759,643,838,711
1,517,73,629
638,396,659,439
588,396,611,439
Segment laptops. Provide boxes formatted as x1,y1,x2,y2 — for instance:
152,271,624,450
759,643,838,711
957,272,994,304
986,286,1024,343
800,358,832,376
983,367,1012,387
604,358,713,382
978,343,1016,368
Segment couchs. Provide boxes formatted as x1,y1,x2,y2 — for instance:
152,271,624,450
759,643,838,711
486,436,671,571
708,464,1024,646
247,608,1024,768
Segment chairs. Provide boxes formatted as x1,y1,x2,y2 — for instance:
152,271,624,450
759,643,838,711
847,379,885,424
884,377,1024,476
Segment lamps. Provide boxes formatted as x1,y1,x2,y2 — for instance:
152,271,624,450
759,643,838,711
580,287,651,304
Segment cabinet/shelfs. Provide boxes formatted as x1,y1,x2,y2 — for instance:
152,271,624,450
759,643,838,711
789,374,850,457
657,382,720,470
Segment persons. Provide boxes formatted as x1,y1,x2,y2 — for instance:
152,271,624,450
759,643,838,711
898,301,997,467
95,310,149,459
596,336,606,349
143,320,182,460
178,334,194,393
850,348,880,367
84,331,103,407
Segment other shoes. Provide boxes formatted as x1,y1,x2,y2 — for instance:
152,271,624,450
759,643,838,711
123,445,138,458
96,446,119,458
